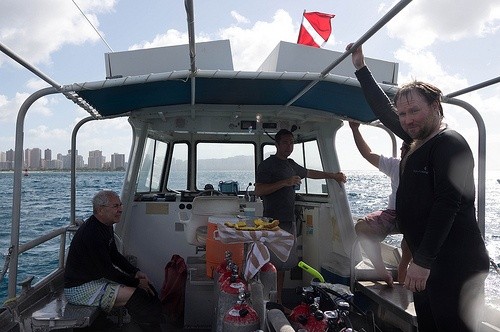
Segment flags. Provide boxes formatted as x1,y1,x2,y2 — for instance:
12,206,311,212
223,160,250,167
297,12,335,48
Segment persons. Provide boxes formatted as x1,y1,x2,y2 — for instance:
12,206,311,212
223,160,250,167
63,191,159,332
345,43,490,332
253,129,347,314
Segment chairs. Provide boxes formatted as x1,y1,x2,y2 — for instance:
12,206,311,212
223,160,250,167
179,196,241,255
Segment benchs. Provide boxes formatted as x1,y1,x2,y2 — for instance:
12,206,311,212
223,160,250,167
356,283,494,332
32,293,123,332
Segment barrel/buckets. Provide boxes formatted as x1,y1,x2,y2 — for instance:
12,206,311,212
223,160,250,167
206,215,244,279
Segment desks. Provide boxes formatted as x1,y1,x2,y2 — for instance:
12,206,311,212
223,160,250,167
217,219,294,280
